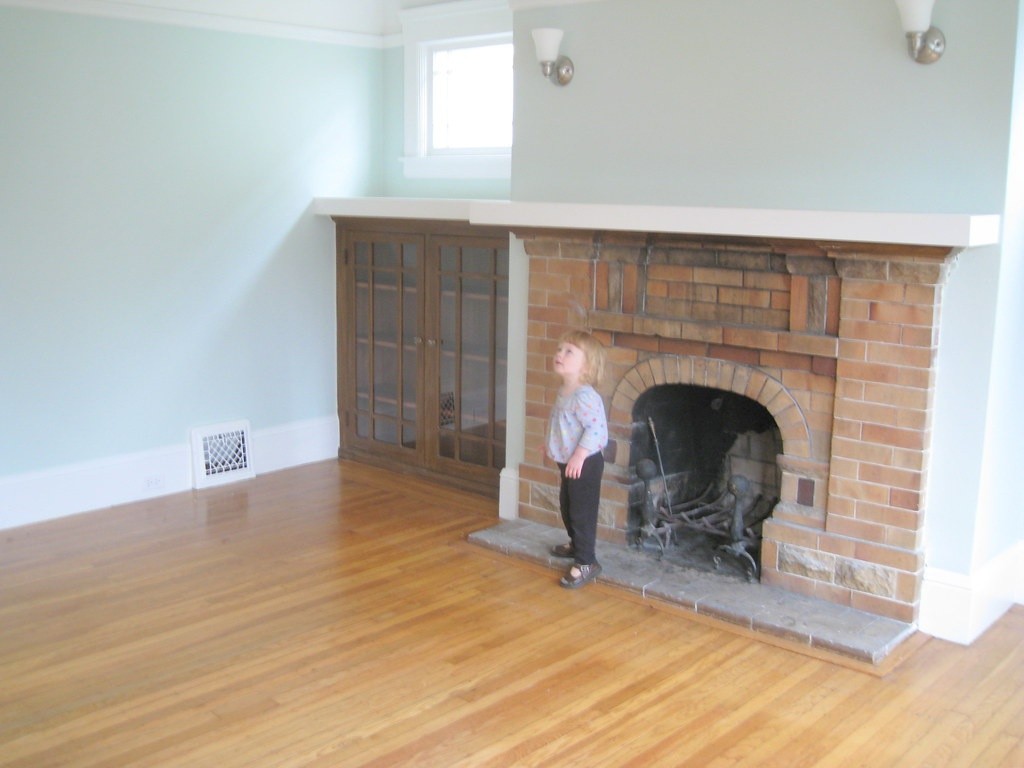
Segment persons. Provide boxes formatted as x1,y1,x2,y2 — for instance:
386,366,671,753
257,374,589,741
544,331,607,590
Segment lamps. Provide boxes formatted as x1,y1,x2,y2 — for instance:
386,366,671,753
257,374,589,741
531,27,574,86
895,0,945,64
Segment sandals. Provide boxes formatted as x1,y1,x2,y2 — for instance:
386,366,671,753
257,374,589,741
560,560,602,589
549,542,576,557
